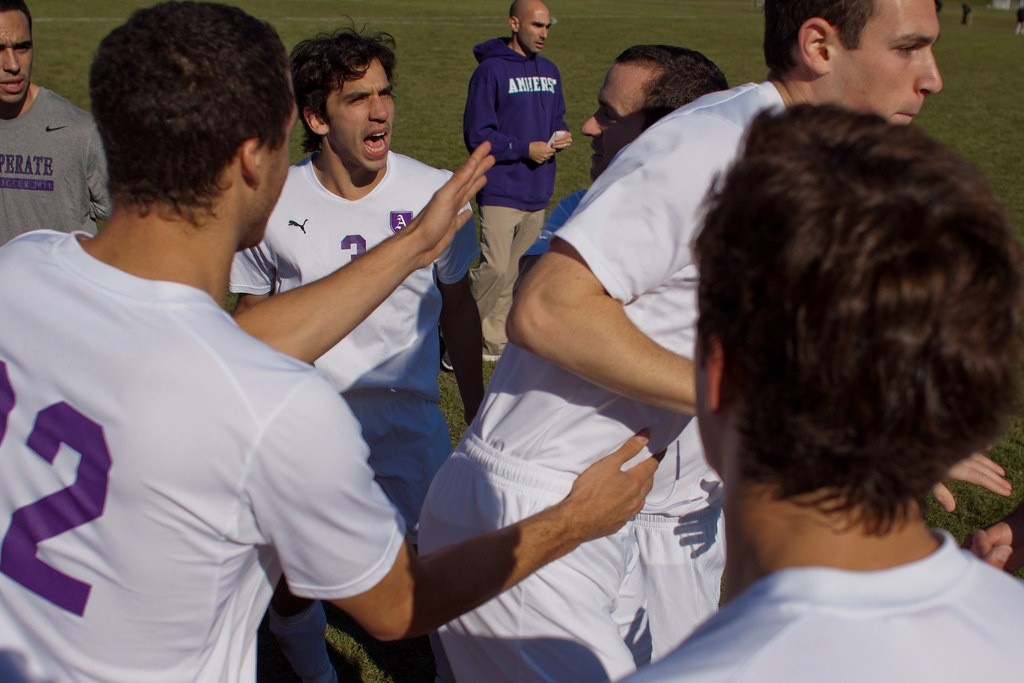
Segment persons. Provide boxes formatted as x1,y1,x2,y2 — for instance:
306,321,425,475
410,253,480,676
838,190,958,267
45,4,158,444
0,0,112,247
230,28,483,683
420,0,1011,683
441,0,573,371
0,1,667,683
935,0,1024,35
513,43,729,295
618,102,1023,683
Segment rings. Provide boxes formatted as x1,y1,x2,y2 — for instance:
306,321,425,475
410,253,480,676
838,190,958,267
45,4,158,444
564,144,567,147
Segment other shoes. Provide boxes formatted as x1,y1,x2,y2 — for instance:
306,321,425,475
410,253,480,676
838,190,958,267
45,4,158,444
442,350,453,371
483,353,501,360
